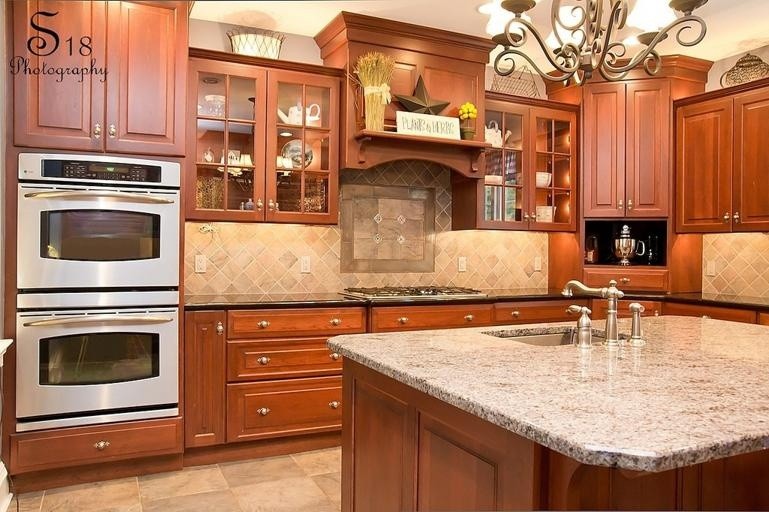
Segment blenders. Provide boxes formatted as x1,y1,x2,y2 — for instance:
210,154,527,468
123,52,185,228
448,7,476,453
639,228,659,265
606,224,636,266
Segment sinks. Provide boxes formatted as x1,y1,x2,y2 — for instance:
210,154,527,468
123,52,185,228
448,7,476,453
478,327,632,346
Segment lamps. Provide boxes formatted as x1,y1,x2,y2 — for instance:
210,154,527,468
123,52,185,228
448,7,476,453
477,0,708,86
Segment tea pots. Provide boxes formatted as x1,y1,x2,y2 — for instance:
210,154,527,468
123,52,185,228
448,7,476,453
277,102,320,125
485,120,512,148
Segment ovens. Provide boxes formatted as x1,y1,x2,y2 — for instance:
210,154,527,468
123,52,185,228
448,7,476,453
15,152,180,434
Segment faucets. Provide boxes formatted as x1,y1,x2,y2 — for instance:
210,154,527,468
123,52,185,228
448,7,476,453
562,280,624,341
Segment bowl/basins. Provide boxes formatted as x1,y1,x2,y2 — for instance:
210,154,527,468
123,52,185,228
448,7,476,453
536,172,552,187
485,175,502,185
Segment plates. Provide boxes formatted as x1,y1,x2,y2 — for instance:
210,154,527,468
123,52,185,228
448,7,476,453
281,139,313,169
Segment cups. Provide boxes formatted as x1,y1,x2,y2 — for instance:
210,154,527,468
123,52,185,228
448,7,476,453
205,95,225,117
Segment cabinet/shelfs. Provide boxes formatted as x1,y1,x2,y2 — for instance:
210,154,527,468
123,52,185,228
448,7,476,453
671,77,769,233
186,47,345,226
663,298,765,325
548,53,715,217
452,91,579,231
2,0,194,158
495,298,589,325
589,297,663,321
313,10,498,181
547,217,703,299
368,305,495,331
182,307,226,466
227,301,369,462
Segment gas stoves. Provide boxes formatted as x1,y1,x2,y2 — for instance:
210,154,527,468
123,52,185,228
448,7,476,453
337,286,488,302
